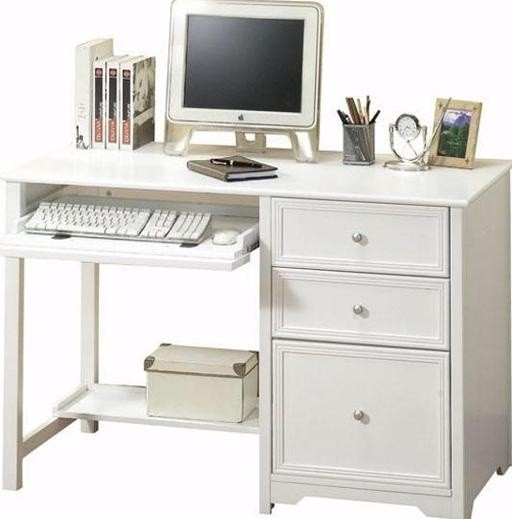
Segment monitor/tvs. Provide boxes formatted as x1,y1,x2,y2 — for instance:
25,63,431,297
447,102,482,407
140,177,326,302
165,0,325,165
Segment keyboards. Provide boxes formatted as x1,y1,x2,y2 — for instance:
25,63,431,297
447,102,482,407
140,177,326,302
25,202,213,245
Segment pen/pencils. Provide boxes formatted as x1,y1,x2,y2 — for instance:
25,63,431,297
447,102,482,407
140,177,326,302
210,159,263,167
337,96,380,163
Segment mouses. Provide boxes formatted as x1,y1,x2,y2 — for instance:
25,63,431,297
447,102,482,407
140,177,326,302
212,226,241,245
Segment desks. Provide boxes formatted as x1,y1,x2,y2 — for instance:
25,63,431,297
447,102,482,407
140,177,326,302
0,139,512,518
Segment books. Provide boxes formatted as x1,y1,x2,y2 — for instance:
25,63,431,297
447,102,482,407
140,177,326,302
120,55,154,150
186,154,278,182
92,53,117,149
74,38,114,149
105,55,135,150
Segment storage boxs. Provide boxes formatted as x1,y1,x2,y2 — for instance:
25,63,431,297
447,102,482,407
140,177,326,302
143,343,262,424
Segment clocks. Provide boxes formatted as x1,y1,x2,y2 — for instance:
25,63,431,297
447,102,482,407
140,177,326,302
383,115,430,171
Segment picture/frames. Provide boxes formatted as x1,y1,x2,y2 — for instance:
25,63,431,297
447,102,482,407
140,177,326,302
426,98,482,169
